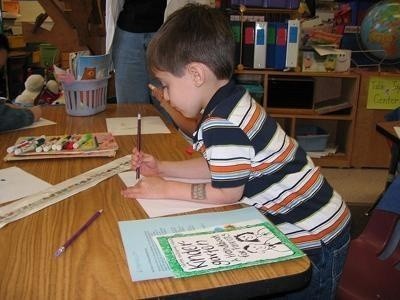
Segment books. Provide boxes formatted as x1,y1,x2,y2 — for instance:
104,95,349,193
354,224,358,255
68,50,110,109
304,29,344,48
313,97,353,115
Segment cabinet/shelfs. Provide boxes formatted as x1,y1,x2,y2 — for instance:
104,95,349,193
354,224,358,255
218,0,400,168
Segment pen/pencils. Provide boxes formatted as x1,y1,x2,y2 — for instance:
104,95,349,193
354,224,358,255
137,114,141,181
54,208,105,257
7,133,96,154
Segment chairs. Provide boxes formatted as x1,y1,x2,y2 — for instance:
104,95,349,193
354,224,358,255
331,173,400,299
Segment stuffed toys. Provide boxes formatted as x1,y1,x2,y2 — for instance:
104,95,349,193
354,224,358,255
13,73,58,107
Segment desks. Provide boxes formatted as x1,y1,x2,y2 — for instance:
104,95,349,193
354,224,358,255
372,119,400,212
1,102,312,299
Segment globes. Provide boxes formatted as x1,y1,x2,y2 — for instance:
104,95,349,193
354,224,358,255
358,0,400,70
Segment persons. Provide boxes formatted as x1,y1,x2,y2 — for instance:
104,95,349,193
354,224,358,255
120,3,353,300
0,33,42,131
105,0,221,128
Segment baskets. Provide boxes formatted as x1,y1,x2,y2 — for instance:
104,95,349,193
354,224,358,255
59,76,111,116
